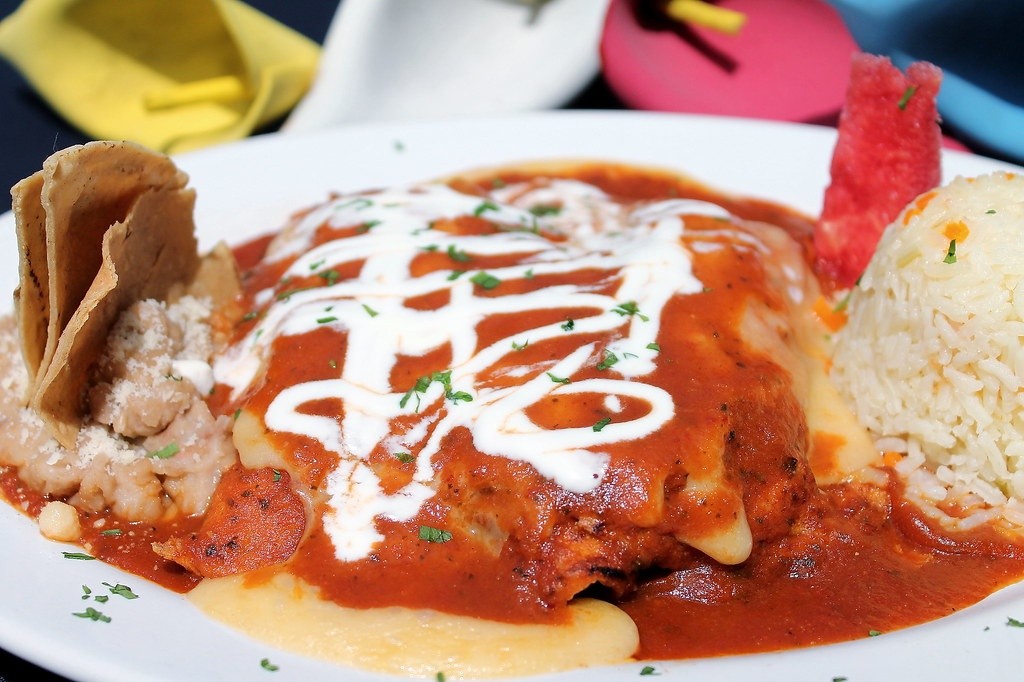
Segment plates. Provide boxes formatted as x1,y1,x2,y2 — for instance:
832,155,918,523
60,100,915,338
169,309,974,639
0,109,1024,682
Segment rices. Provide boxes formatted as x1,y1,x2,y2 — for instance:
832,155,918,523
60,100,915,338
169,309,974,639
825,171,1024,530
